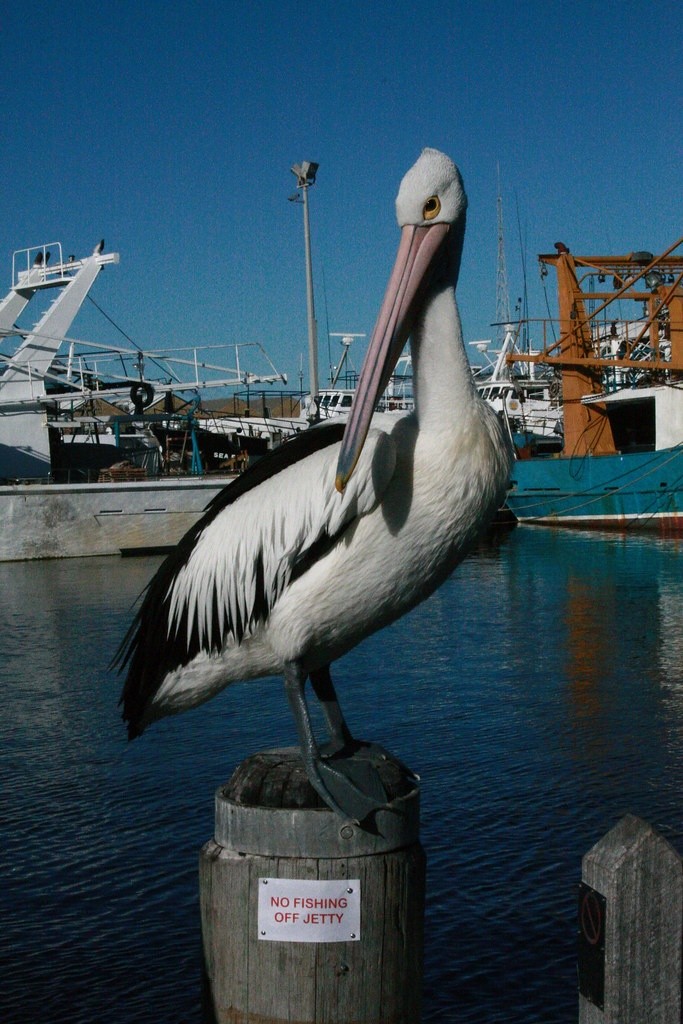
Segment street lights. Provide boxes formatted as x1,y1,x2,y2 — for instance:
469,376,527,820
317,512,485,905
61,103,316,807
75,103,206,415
289,160,320,424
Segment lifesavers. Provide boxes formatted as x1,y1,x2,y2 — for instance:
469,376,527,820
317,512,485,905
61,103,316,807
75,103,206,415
130,382,156,407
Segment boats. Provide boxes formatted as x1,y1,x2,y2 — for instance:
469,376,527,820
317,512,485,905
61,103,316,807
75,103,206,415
0,239,385,561
305,322,668,462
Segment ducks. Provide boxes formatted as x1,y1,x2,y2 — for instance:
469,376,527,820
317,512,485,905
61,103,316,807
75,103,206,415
102,147,517,824
